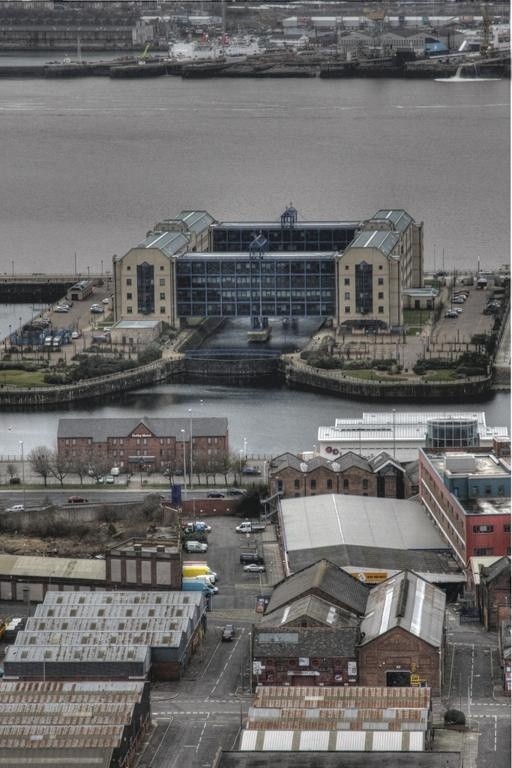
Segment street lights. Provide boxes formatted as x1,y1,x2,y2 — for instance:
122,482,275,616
248,632,252,696
181,408,193,501
19,440,26,503
239,438,248,465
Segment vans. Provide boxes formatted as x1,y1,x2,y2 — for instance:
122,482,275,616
240,553,264,564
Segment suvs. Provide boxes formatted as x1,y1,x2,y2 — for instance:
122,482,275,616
185,522,212,533
185,540,208,553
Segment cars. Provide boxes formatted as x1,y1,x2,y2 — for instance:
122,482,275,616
481,288,506,315
244,563,265,572
445,290,470,318
243,467,261,475
222,624,234,641
5,504,25,513
206,488,244,498
68,496,88,502
12,298,109,347
95,475,114,483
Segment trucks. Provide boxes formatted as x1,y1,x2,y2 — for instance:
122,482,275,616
88,465,95,476
181,561,219,597
235,521,266,533
111,468,121,475
